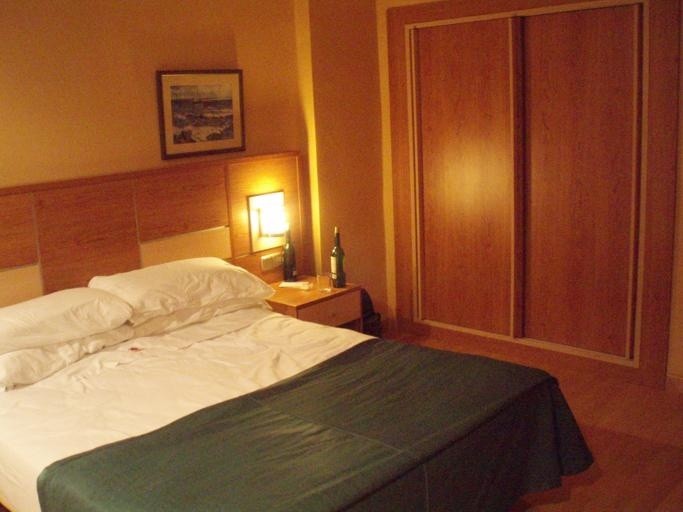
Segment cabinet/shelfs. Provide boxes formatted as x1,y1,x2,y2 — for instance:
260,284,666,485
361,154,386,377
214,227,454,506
386,3,633,366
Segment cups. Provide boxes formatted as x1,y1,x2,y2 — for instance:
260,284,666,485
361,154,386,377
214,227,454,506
316,272,331,293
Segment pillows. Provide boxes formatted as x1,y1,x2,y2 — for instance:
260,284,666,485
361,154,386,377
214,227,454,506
87,258,276,333
0,287,133,391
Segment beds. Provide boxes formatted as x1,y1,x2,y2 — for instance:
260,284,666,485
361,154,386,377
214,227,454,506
0,258,593,512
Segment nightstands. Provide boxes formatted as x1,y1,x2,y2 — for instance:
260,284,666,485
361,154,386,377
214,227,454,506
261,274,364,334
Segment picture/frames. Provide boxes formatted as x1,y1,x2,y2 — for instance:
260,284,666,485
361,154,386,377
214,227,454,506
155,67,244,161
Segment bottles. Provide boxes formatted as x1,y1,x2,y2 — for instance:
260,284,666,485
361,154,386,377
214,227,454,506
283,229,297,283
329,226,346,289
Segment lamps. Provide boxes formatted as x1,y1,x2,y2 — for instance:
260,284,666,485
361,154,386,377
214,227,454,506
245,190,290,253
